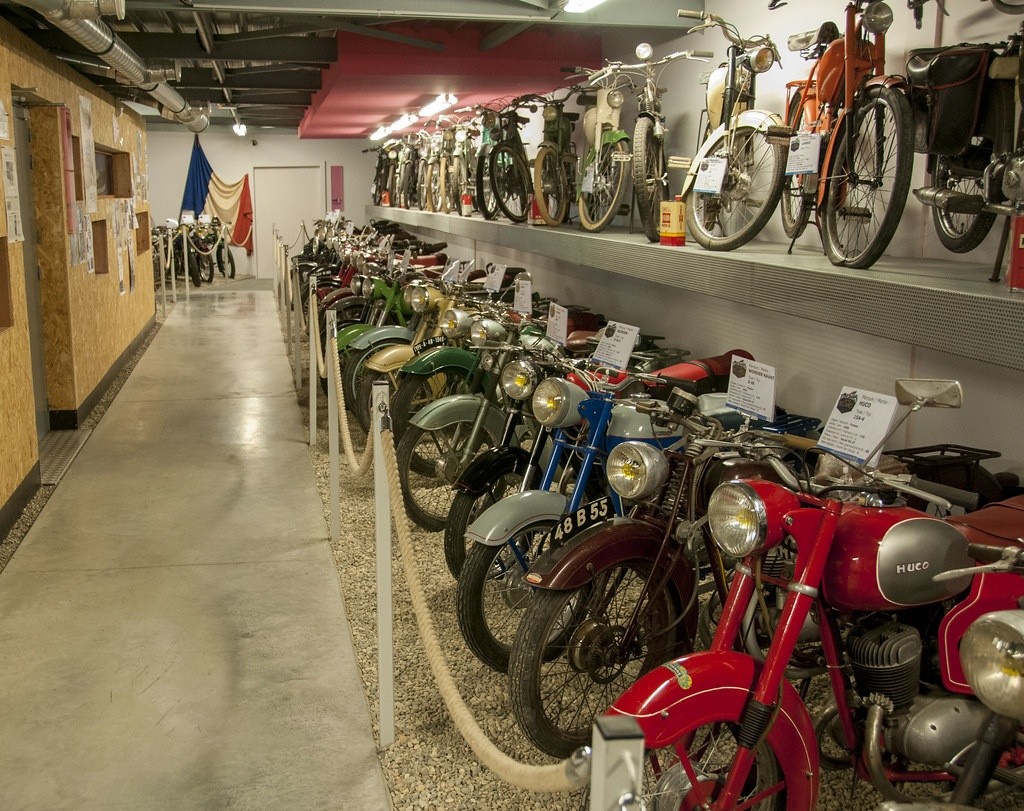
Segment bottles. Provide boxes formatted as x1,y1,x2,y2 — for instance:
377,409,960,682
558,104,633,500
461,192,472,217
659,195,686,246
381,189,390,206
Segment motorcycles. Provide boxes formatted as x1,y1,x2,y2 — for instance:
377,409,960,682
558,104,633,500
371,0,1024,291
150,218,237,290
276,210,1024,811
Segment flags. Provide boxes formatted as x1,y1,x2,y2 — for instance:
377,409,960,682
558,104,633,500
178,134,254,256
